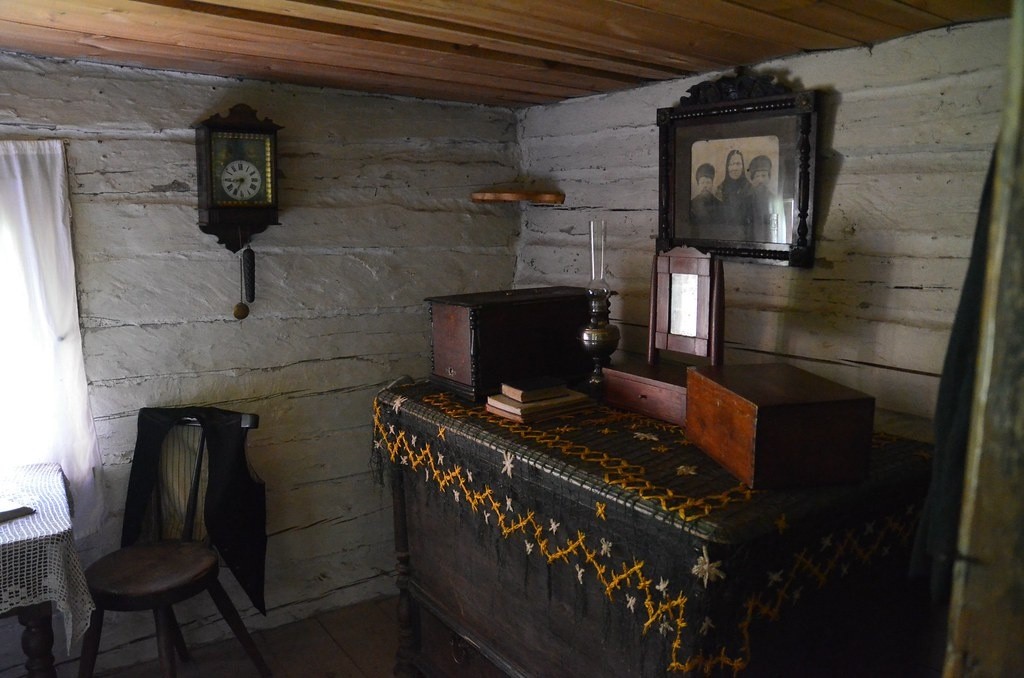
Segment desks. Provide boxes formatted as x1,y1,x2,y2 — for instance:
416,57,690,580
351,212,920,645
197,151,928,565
0,463,96,678
367,381,947,678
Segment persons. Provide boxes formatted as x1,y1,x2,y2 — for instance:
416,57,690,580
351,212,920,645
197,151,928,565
716,150,753,239
690,164,728,241
742,156,783,244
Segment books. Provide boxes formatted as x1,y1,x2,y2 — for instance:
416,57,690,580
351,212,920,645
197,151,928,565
500,380,570,403
488,391,588,415
485,404,598,423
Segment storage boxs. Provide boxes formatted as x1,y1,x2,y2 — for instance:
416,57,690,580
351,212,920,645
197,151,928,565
686,362,876,491
602,359,698,427
423,285,619,402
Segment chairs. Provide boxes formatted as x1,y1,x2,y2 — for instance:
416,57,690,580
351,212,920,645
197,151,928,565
80,407,267,678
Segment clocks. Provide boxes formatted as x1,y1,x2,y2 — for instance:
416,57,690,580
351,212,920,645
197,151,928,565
192,103,285,320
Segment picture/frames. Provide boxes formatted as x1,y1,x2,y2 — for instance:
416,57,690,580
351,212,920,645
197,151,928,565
655,67,826,268
